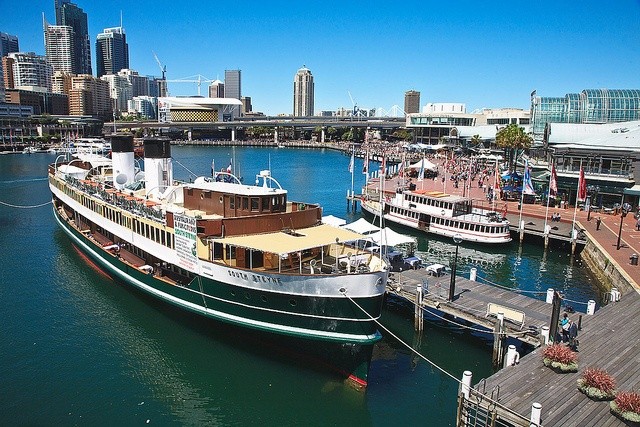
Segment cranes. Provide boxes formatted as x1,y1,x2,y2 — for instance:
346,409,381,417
156,74,213,97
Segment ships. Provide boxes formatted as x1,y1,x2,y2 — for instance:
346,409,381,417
362,189,515,246
49,136,111,153
47,133,391,396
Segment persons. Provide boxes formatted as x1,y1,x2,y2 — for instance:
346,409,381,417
596,217,601,230
636,217,640,231
494,194,497,200
567,320,578,349
517,197,522,210
442,159,494,202
551,212,561,223
559,312,570,343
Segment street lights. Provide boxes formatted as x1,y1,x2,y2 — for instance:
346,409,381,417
449,233,463,301
613,202,633,250
586,184,600,221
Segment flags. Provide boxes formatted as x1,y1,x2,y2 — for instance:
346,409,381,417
348,153,354,172
362,155,367,174
398,160,405,179
382,157,385,174
210,159,214,176
418,159,424,181
495,161,500,189
227,162,232,179
523,159,536,195
577,163,587,202
550,160,557,196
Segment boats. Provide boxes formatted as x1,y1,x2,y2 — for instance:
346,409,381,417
24,145,52,153
